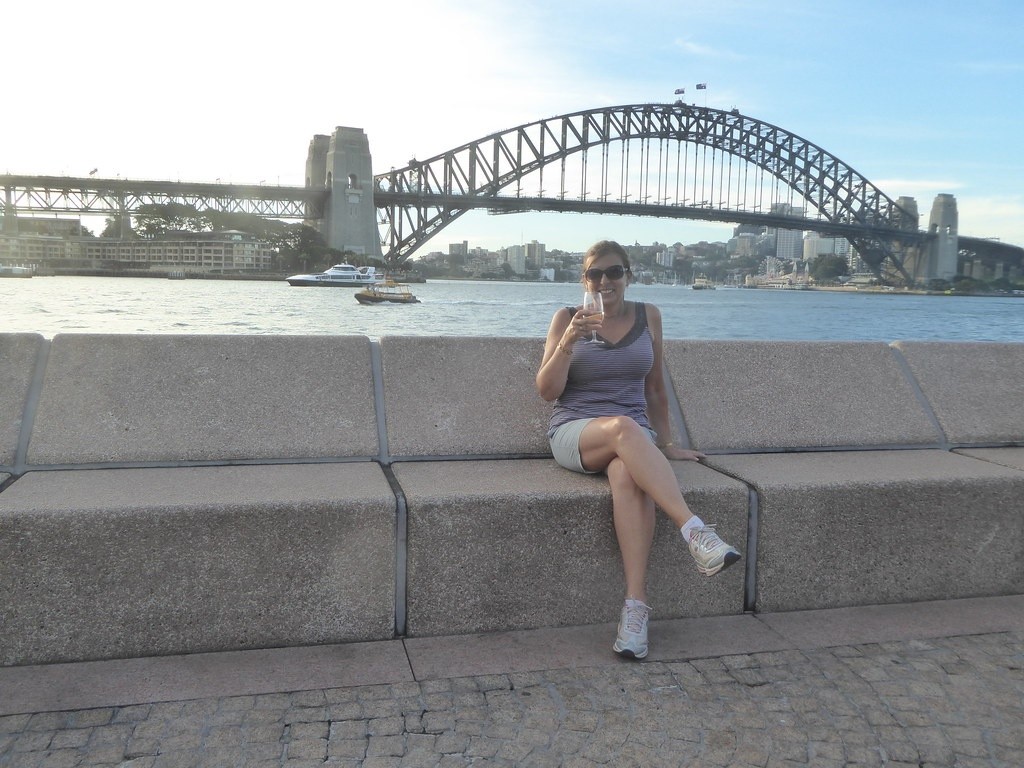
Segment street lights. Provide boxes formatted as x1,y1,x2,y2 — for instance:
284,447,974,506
259,178,266,214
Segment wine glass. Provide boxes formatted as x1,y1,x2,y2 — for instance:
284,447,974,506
583,291,604,346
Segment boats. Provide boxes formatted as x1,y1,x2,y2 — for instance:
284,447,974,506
284,261,387,288
353,276,422,305
691,272,716,291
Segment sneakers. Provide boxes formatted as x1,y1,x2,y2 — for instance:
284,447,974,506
688,524,742,577
612,597,652,659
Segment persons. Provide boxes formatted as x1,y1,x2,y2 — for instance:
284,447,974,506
534,238,744,662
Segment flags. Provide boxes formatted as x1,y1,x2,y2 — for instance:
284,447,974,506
696,82,707,89
675,87,685,94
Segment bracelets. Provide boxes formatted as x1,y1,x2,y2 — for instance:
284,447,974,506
656,442,674,449
557,343,575,356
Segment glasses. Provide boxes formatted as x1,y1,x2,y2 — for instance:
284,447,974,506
582,265,628,284
